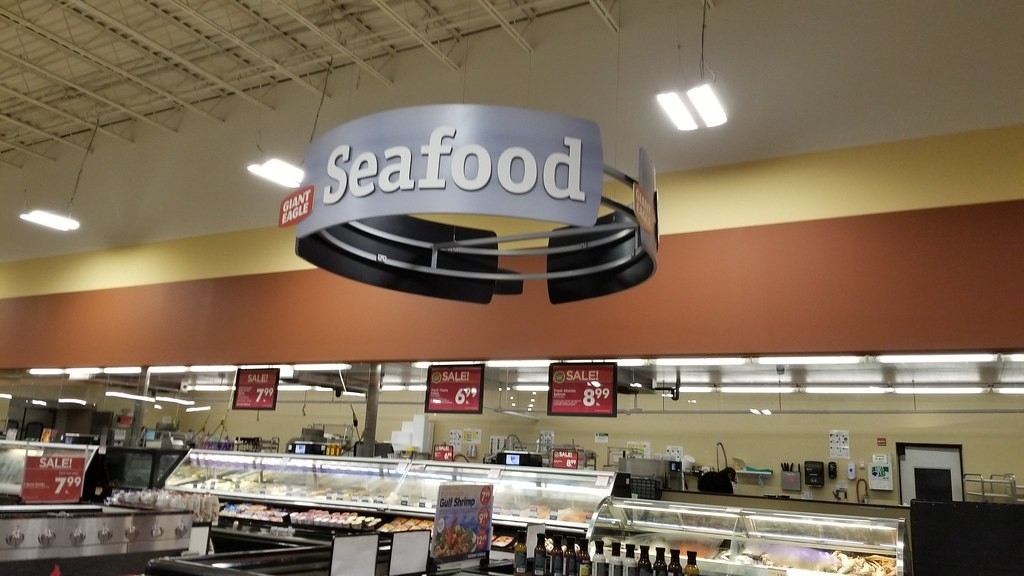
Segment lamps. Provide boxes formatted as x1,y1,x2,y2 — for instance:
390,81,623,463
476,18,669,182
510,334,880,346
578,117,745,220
248,42,333,190
678,383,1024,396
655,0,727,130
20,95,99,232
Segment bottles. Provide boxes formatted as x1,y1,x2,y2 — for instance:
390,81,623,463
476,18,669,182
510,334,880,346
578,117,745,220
684,550,699,576
514,530,528,573
591,540,605,576
200,436,260,452
563,536,577,576
576,538,591,576
623,544,637,576
667,548,682,576
533,533,546,576
652,547,667,576
550,535,563,576
638,545,651,576
608,542,623,576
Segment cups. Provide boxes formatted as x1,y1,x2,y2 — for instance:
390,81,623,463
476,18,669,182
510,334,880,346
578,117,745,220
289,509,375,531
682,454,695,471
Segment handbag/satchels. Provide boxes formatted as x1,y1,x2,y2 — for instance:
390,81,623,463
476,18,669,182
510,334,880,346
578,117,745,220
697,442,738,495
